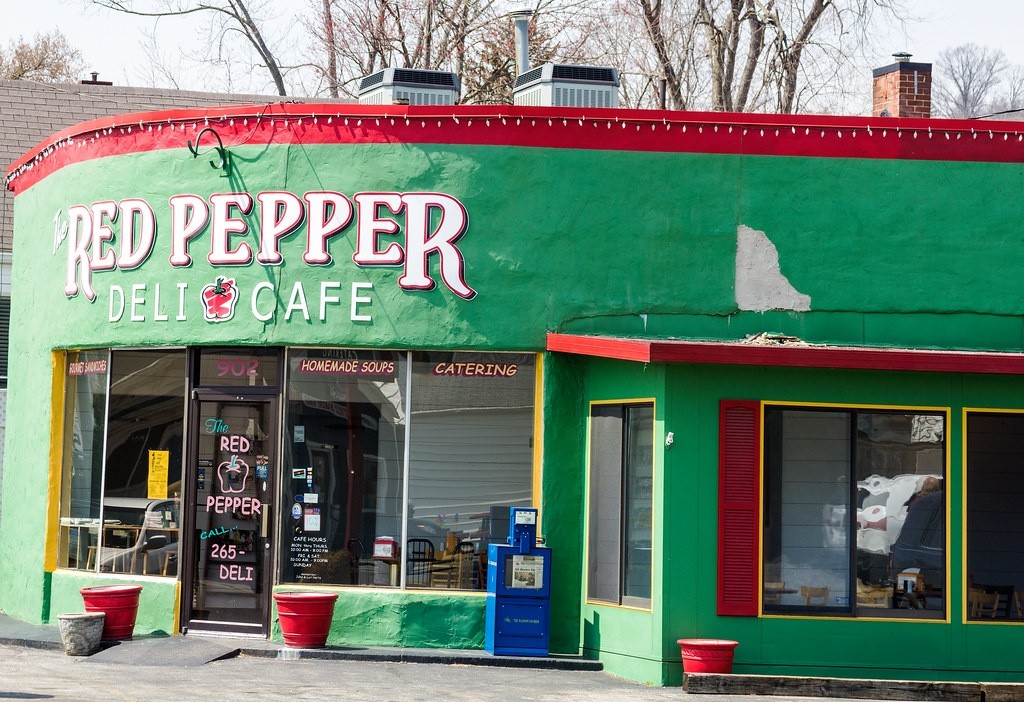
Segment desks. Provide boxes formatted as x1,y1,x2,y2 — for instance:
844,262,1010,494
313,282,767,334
764,588,798,594
58,523,179,574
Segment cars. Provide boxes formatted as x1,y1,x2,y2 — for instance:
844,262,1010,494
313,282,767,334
89,495,182,575
887,492,945,594
821,471,942,560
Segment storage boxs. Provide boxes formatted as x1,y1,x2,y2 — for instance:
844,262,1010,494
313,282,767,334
374,541,399,558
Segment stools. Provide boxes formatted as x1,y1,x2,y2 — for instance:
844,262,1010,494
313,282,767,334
163,551,178,576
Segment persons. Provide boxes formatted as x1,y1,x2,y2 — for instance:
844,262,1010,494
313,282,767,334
906,477,940,513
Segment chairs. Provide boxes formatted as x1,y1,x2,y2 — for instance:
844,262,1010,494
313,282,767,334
447,541,475,589
400,538,435,587
968,591,1000,618
86,545,97,570
799,586,830,605
346,538,370,586
764,582,785,605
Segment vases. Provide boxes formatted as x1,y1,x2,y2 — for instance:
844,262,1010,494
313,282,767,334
79,583,144,640
676,638,739,674
272,591,340,650
57,611,107,656
